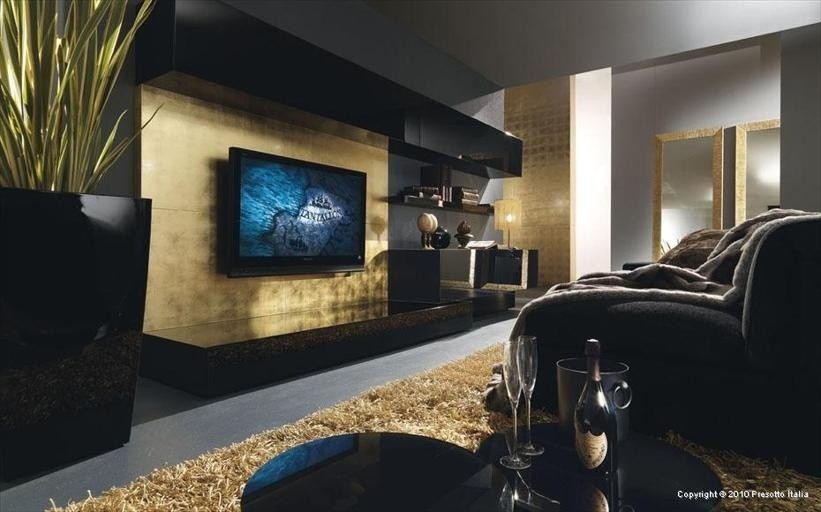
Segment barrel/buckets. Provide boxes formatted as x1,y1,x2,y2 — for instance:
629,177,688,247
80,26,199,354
556,358,633,433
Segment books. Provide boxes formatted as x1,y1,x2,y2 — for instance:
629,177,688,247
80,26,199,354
458,240,497,249
443,186,479,205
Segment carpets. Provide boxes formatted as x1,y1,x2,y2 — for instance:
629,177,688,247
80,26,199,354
44,340,821,512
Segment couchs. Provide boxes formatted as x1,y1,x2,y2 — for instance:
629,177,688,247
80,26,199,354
488,206,821,478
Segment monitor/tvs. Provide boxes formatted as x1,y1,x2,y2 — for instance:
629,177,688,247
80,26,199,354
228,147,367,276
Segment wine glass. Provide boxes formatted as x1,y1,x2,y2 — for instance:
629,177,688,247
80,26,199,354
516,334,546,458
498,338,532,470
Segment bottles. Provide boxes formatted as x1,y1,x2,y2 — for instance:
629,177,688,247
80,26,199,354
431,225,451,249
573,338,620,478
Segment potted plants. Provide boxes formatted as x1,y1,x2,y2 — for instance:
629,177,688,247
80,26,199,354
0,0,165,492
